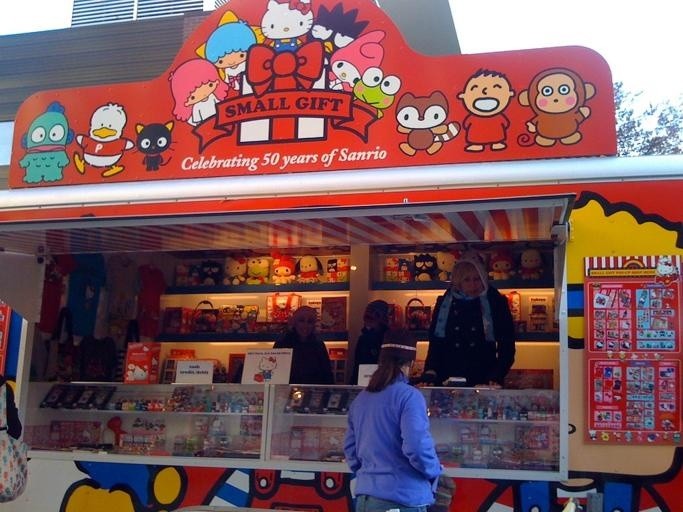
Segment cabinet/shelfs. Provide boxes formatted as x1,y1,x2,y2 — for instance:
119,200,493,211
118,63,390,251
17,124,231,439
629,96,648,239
160,245,351,384
265,385,560,481
368,241,559,390
23,378,269,470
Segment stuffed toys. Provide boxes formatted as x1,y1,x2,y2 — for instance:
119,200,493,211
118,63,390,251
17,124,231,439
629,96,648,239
519,249,544,279
223,255,246,285
438,252,461,282
296,256,324,284
247,258,269,284
176,260,222,286
489,253,514,280
271,252,296,285
415,254,438,281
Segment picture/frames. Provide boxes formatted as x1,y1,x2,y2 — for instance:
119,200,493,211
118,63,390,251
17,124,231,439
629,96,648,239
228,353,246,382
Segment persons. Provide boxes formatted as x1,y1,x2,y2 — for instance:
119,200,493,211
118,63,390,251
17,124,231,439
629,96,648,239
344,332,443,512
274,306,332,385
1,375,28,503
414,252,516,389
346,300,408,385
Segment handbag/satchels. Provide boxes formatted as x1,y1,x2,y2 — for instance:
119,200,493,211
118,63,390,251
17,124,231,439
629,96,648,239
0,386,30,501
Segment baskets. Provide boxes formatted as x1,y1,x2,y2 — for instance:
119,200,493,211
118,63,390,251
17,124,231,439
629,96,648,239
405,299,426,329
191,300,217,332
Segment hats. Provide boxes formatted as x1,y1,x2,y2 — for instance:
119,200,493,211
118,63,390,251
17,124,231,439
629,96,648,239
380,331,419,353
364,299,395,324
